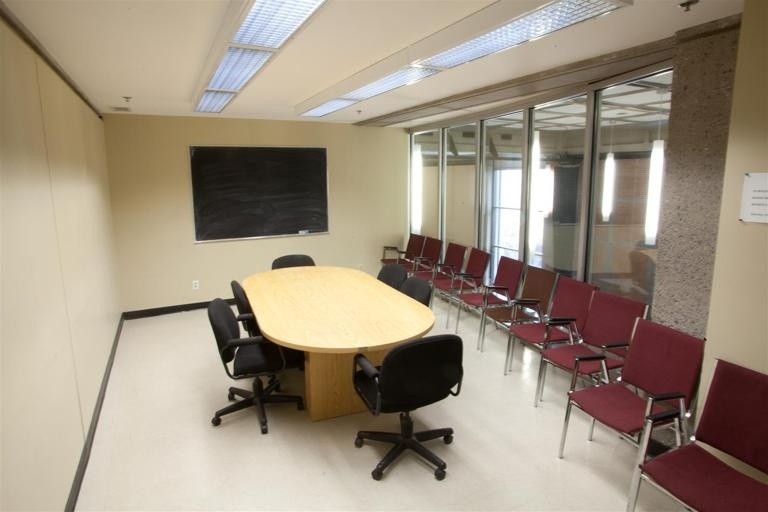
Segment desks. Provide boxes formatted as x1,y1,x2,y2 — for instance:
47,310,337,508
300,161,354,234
241,266,435,421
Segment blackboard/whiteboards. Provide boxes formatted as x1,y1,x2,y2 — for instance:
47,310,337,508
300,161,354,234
189,145,331,244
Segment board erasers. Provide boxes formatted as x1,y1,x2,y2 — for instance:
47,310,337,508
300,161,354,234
298,230,309,235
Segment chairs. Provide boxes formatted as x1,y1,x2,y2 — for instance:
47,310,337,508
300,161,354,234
350,334,463,482
624,355,767,512
503,274,600,401
479,264,558,371
380,233,425,266
415,242,467,283
434,246,491,329
272,254,315,270
532,288,652,410
556,316,705,461
205,298,304,435
231,280,308,391
455,255,527,348
399,236,442,276
